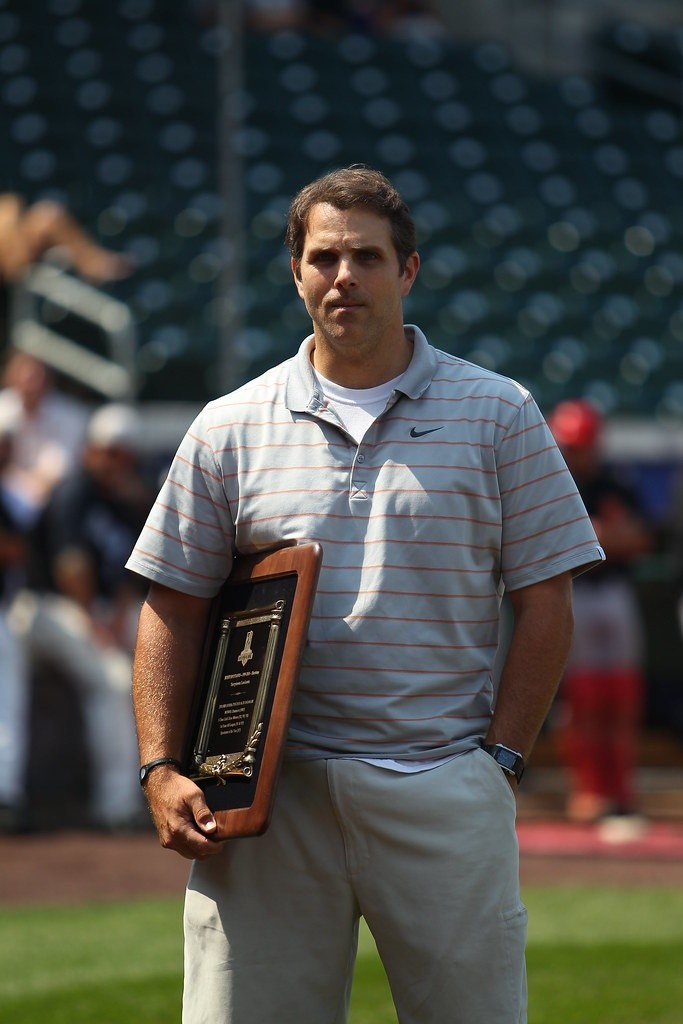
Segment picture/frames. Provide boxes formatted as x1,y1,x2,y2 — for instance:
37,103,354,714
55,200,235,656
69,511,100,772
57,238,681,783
179,544,323,843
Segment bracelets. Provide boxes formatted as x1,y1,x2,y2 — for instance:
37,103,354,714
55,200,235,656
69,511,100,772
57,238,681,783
140,759,181,788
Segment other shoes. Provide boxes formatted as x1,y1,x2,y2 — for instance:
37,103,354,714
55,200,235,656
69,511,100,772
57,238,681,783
569,793,644,847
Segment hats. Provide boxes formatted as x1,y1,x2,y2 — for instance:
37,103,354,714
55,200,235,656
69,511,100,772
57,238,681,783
86,399,146,457
549,398,596,452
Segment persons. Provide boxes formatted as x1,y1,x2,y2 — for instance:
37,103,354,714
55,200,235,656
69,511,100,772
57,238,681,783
116,161,612,1024
538,393,653,831
0,191,168,841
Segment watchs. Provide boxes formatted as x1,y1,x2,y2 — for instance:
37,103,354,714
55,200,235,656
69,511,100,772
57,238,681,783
482,743,526,787
